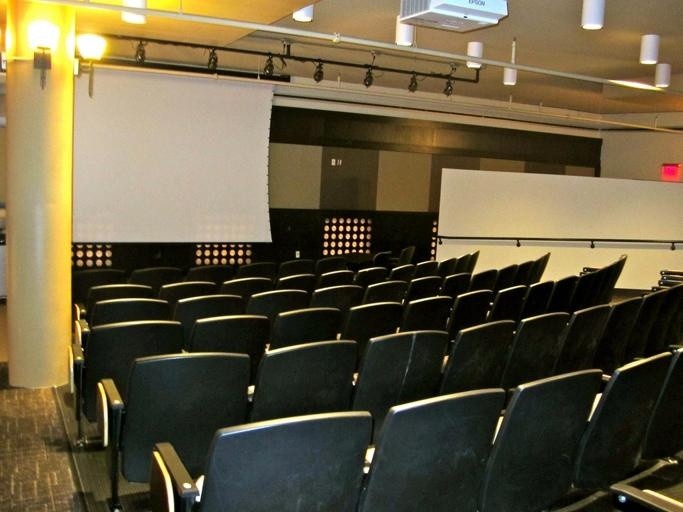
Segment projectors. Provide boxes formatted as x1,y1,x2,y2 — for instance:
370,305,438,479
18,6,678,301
399,0,508,34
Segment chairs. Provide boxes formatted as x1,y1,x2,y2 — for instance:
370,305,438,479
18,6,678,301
73,245,682,508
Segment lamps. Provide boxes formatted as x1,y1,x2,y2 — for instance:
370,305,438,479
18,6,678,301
314,62,324,82
30,20,57,87
443,80,453,95
122,1,148,25
408,74,419,92
395,15,415,47
74,34,106,100
466,42,484,68
655,64,671,87
207,49,218,72
640,35,658,64
580,0,605,31
264,54,276,79
364,69,373,88
503,67,519,86
135,40,147,64
291,5,314,22
